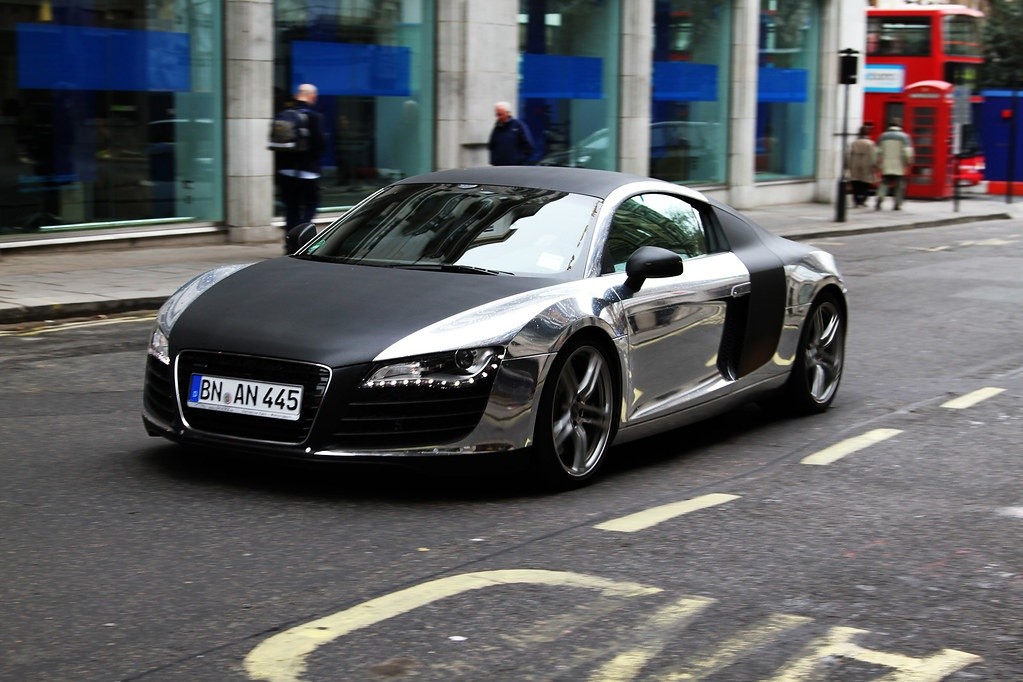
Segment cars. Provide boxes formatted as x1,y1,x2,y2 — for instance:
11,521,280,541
539,122,725,183
91,119,216,184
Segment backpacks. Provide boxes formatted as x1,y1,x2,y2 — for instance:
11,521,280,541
269,110,308,155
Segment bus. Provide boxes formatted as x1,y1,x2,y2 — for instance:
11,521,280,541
666,4,987,188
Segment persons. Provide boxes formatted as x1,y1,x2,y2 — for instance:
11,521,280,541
278,83,333,255
874,116,915,210
488,103,535,165
845,124,880,208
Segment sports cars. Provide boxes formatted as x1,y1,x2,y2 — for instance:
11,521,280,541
142,166,849,491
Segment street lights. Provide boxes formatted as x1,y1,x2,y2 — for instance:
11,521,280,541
835,48,859,220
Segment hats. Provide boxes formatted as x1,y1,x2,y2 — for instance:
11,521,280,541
858,124,870,136
889,118,904,128
